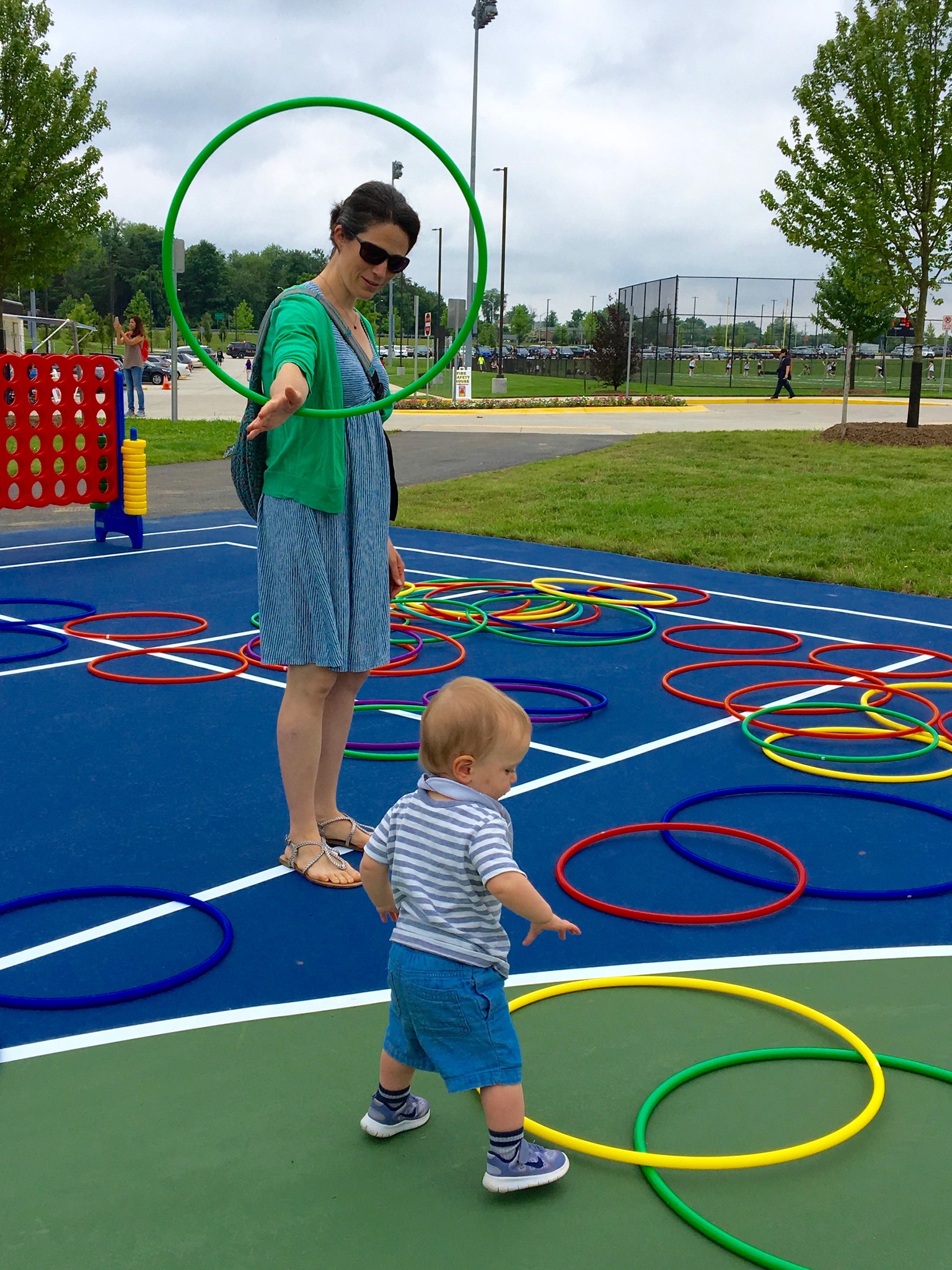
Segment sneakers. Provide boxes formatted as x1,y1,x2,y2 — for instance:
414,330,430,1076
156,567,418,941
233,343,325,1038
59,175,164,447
124,409,135,416
135,409,146,416
482,1138,570,1193
360,1092,430,1138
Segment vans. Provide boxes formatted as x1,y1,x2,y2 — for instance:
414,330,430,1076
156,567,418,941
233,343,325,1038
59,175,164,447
538,341,553,345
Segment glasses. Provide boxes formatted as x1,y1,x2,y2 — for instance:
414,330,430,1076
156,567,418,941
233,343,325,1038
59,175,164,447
335,222,410,273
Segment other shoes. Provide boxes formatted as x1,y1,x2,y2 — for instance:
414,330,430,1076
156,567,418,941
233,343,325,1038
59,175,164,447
770,395,778,398
788,395,795,398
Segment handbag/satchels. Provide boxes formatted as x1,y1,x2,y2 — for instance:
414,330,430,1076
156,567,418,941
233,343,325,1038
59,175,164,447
222,288,384,524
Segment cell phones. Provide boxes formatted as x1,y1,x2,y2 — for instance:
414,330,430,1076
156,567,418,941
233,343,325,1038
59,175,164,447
115,316,119,323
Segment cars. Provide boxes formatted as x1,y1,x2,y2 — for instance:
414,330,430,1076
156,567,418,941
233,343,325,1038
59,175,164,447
53,346,217,393
383,345,389,353
392,345,434,358
639,343,847,360
855,346,875,359
375,346,388,358
471,343,605,358
890,343,952,359
459,346,474,358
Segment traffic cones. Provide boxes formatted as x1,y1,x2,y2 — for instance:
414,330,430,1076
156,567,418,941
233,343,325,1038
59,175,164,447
162,374,172,390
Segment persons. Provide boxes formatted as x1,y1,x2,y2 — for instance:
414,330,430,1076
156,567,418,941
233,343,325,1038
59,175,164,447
924,360,937,382
757,358,763,376
113,316,146,417
380,357,386,370
360,676,581,1194
743,358,750,378
246,181,421,889
725,357,734,379
689,357,697,378
243,360,251,381
822,360,837,378
803,364,810,376
457,345,591,376
874,365,884,380
25,349,53,380
770,349,795,399
217,348,224,368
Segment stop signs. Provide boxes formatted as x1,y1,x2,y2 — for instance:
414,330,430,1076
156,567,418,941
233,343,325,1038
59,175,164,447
424,313,431,335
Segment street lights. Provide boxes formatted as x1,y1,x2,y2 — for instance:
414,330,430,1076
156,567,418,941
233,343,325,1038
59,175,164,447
482,299,494,325
388,161,404,369
692,296,699,347
110,255,118,340
431,227,443,367
666,303,672,346
545,299,550,348
374,299,383,325
539,311,549,345
492,167,506,380
590,295,597,343
770,299,778,348
759,305,766,346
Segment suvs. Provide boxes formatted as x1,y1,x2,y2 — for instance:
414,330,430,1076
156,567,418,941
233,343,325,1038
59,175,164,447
226,342,258,359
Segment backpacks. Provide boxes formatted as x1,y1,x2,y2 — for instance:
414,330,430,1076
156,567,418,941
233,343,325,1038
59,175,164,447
126,332,149,362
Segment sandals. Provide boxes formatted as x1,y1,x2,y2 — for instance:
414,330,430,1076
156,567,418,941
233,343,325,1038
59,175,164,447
279,834,363,888
316,812,376,852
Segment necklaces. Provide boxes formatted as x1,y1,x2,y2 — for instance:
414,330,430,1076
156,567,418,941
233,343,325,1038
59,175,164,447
318,274,357,329
